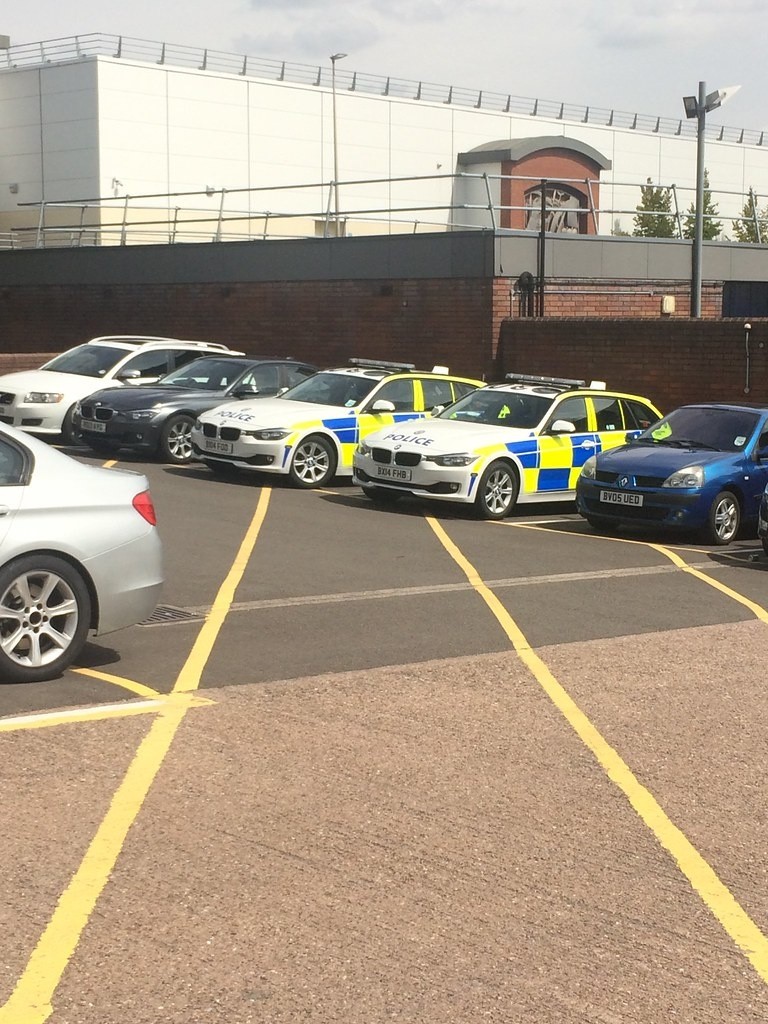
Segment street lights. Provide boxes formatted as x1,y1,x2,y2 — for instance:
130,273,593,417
682,80,723,318
330,52,349,243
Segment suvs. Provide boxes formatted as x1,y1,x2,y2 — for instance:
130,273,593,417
0,334,259,448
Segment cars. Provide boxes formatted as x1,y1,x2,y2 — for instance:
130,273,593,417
350,372,675,521
191,357,511,490
0,419,166,685
572,402,768,545
70,352,338,465
